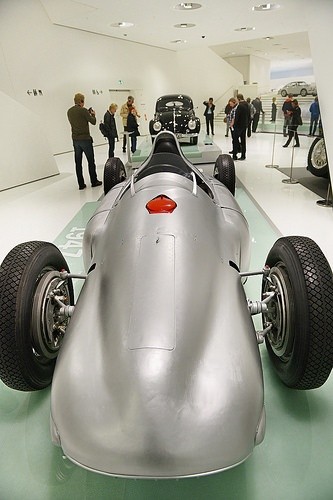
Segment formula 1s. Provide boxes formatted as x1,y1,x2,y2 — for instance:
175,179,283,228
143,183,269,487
0,131,333,479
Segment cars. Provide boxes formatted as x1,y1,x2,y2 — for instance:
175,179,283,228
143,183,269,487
148,94,200,145
278,81,317,97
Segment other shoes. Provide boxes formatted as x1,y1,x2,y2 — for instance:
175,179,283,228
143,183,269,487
292,144,300,147
79,184,86,190
232,157,245,160
224,134,227,137
228,150,236,154
282,145,287,147
91,180,101,187
211,133,214,135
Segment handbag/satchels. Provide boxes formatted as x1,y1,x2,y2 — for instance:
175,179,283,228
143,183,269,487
99,123,107,137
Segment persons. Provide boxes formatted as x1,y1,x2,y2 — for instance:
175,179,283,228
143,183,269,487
104,103,119,159
224,94,265,160
67,94,102,190
308,96,320,136
203,97,216,135
271,93,303,148
120,96,141,153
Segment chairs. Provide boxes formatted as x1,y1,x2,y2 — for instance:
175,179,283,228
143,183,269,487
137,133,202,184
